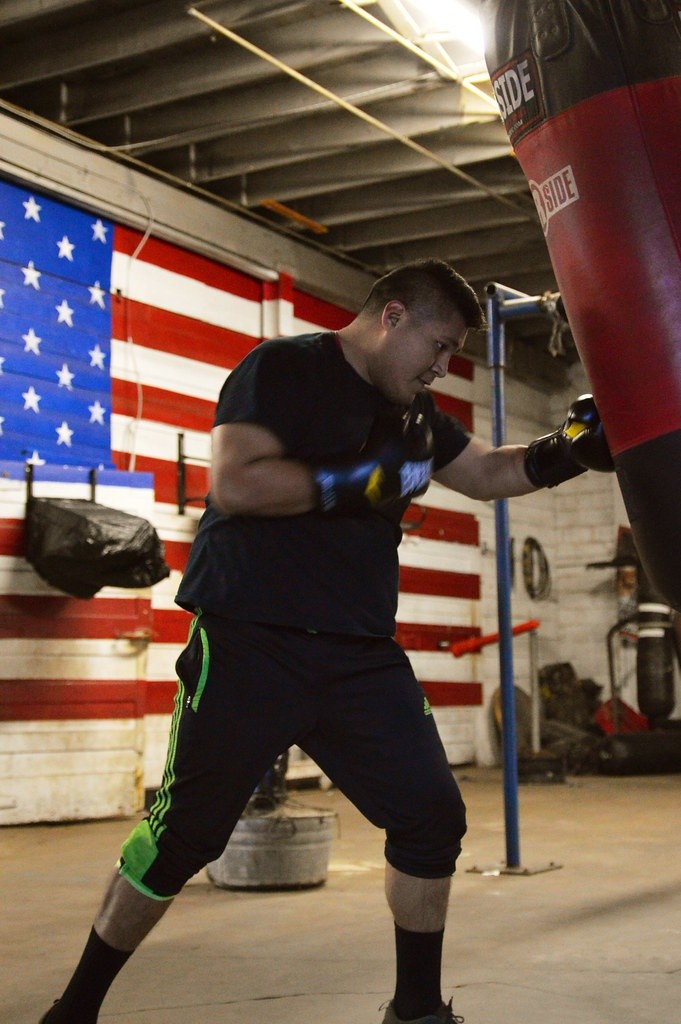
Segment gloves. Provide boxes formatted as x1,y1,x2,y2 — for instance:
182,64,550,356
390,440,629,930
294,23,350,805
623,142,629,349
313,406,433,518
526,395,614,487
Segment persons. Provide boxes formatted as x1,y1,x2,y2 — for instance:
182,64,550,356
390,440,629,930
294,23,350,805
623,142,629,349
36,260,616,1024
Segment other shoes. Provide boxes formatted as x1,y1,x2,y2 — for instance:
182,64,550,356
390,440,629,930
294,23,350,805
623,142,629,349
383,1002,464,1024
